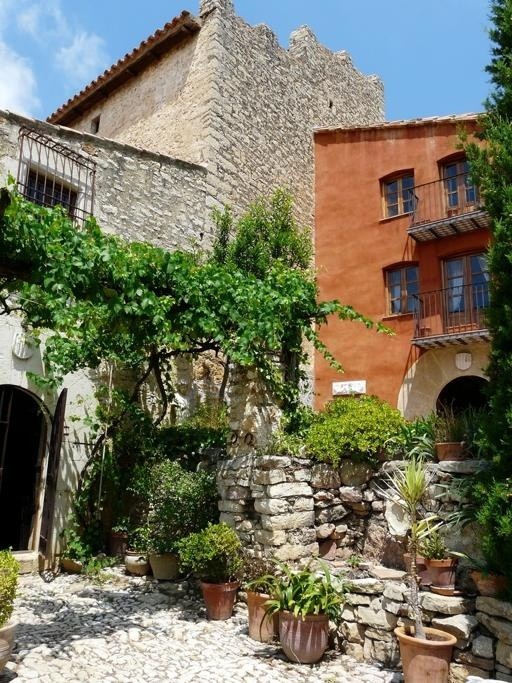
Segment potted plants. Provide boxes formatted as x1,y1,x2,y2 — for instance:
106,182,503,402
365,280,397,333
245,552,352,663
113,460,217,579
239,549,289,641
373,454,458,683
170,521,246,620
404,519,456,595
0,546,22,677
